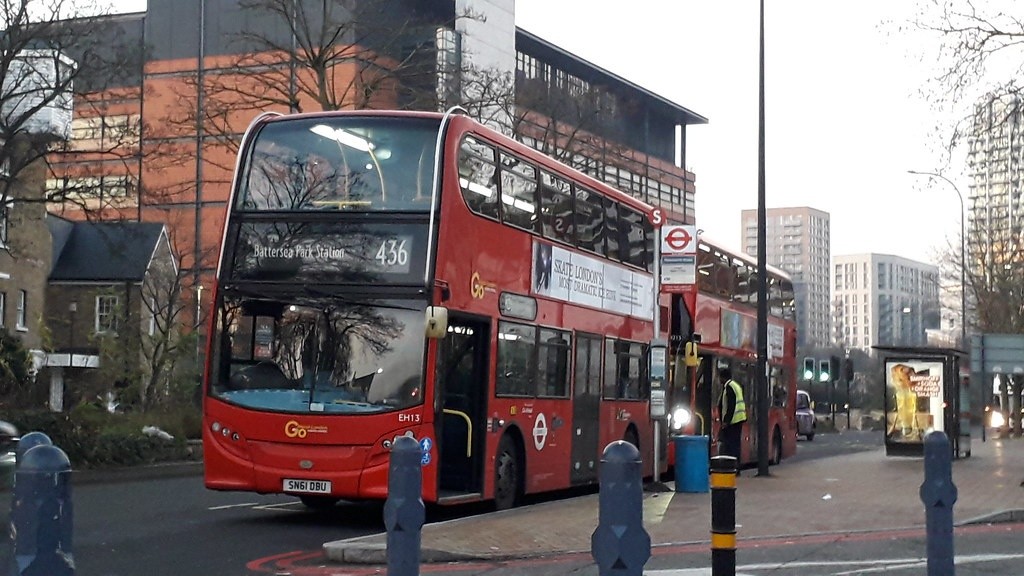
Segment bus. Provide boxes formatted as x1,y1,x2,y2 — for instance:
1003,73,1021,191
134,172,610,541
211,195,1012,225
199,108,669,516
667,233,800,472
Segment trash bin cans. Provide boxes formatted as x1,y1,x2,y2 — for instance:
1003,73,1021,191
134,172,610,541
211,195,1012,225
672,434,710,494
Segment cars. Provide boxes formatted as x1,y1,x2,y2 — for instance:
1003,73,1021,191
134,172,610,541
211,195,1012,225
794,389,816,440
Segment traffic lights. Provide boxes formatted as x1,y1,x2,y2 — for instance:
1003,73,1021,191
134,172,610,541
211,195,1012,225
803,357,815,381
818,360,830,383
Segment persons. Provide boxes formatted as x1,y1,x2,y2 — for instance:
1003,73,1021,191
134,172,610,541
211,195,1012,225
715,366,749,479
988,394,1007,442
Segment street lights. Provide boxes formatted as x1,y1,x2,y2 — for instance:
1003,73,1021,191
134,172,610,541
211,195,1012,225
906,170,967,344
65,296,78,369
